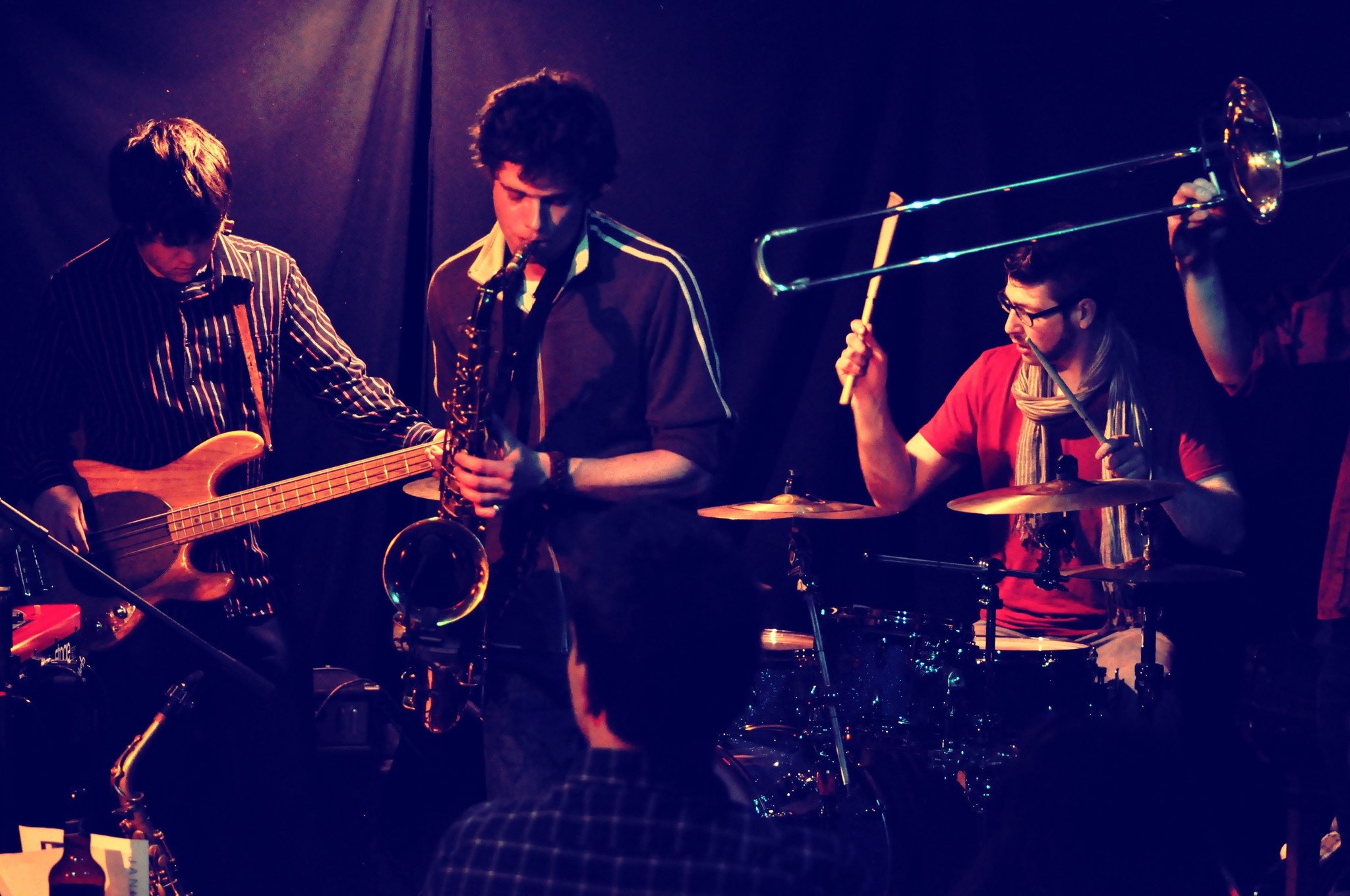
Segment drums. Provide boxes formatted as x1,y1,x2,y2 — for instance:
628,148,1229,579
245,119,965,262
943,630,1106,806
809,604,960,700
714,653,874,820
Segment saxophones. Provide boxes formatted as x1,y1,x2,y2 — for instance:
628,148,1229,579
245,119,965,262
100,679,205,896
384,238,546,742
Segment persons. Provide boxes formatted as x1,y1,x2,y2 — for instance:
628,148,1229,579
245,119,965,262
36,115,454,892
418,549,813,894
1165,175,1350,896
831,229,1242,703
422,64,741,786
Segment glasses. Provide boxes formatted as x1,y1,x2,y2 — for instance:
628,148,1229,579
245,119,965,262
999,295,1091,326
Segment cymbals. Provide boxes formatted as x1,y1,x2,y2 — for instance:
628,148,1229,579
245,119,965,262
944,474,1196,516
1059,555,1250,589
694,496,897,523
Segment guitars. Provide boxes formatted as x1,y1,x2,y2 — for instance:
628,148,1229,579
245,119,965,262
6,428,450,671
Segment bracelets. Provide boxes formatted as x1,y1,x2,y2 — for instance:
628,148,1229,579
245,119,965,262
546,449,571,496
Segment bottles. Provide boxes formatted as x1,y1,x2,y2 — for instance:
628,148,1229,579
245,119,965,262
48,789,107,896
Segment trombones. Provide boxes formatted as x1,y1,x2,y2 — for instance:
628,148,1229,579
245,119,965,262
755,66,1349,296
